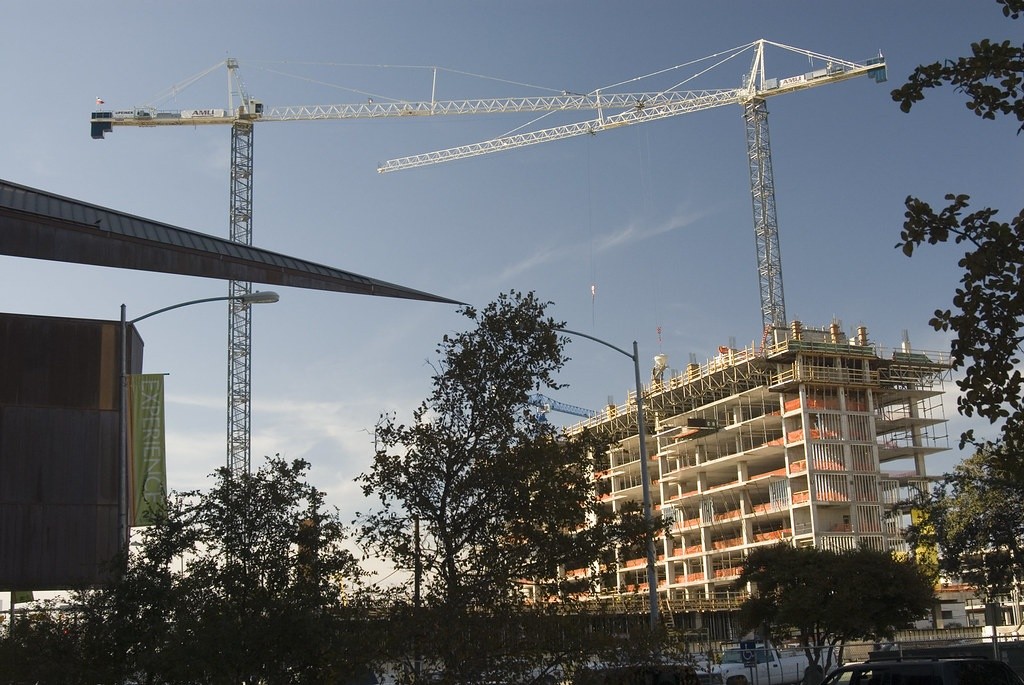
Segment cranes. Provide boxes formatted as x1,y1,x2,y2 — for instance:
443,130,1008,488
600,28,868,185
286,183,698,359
89,51,751,598
528,393,603,427
375,39,889,344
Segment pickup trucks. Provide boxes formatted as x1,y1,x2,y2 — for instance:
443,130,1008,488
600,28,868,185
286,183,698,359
695,643,837,685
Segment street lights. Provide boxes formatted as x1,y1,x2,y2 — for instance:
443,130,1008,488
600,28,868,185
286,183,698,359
117,291,279,580
517,321,662,662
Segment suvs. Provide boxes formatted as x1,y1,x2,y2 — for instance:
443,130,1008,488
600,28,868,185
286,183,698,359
819,656,1024,685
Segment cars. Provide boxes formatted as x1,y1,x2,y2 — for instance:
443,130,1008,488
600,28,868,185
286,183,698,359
482,659,563,685
